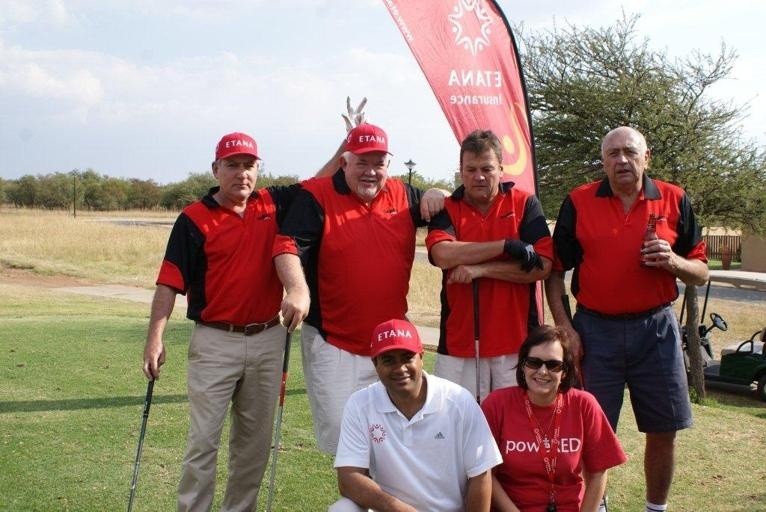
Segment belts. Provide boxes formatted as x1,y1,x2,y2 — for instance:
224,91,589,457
195,315,279,338
575,301,673,324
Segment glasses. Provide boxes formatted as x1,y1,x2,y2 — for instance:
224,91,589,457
521,355,571,374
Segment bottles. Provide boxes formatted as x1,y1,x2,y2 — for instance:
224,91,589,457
638,214,661,266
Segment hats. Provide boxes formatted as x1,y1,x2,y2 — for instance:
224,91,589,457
345,123,394,158
213,132,263,165
369,318,424,360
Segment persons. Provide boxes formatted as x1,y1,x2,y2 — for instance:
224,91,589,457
272,122,451,457
424,129,554,403
548,125,710,512
143,95,373,512
328,320,501,512
485,325,625,512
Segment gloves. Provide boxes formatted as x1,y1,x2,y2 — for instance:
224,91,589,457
502,238,545,274
340,95,370,136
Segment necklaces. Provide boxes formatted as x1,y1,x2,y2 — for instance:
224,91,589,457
535,407,557,440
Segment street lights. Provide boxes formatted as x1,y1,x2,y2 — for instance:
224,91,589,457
70,168,80,218
404,159,417,185
